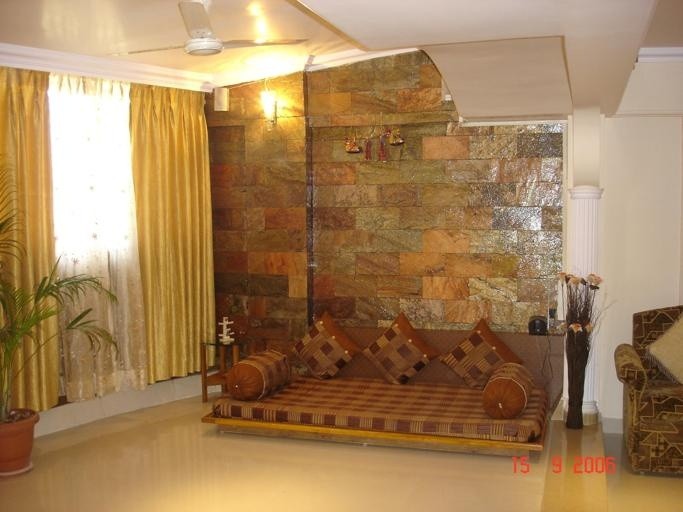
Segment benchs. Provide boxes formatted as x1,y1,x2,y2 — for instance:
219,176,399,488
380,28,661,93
202,326,564,464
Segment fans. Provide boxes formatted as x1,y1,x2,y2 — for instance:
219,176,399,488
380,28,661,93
109,0,309,57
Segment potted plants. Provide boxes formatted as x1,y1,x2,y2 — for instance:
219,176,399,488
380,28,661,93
0,152,121,477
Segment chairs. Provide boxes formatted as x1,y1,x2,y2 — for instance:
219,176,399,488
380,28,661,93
615,304,683,476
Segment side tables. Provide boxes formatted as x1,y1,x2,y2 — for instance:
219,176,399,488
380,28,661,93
200,337,263,402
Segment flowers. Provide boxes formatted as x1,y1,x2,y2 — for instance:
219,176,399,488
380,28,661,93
558,271,618,343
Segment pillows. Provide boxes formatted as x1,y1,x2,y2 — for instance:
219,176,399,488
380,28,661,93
292,310,524,390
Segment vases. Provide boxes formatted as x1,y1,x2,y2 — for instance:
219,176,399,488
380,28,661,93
565,343,588,429
